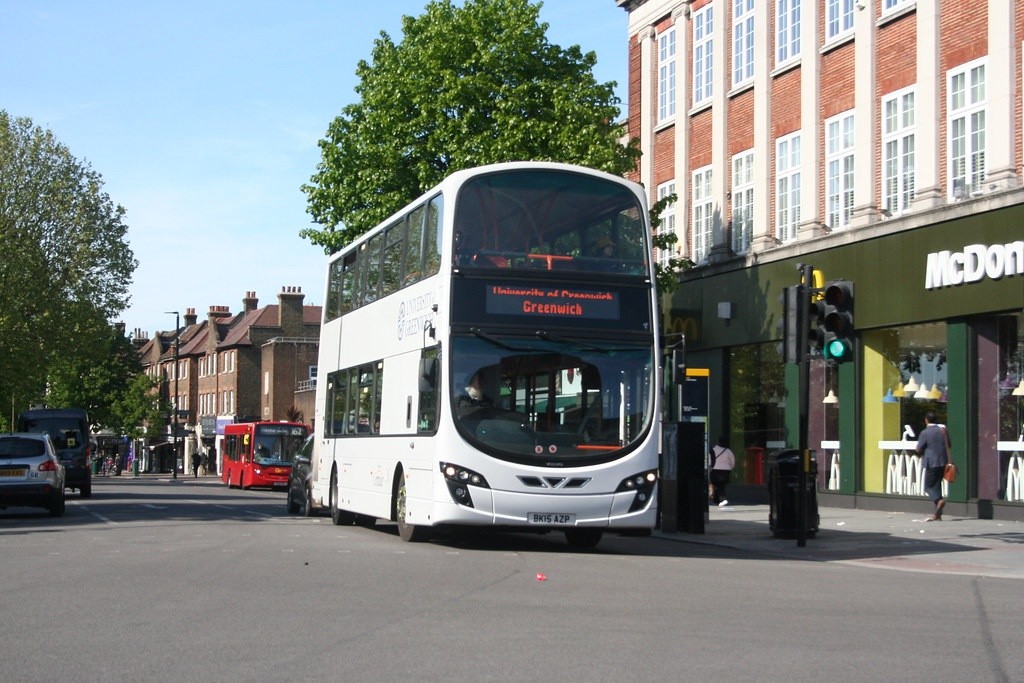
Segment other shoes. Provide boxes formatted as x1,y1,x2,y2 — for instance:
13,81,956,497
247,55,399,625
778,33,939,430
928,514,941,522
718,500,728,506
937,500,944,510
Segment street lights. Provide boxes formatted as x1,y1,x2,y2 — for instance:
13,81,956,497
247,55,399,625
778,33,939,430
165,311,180,479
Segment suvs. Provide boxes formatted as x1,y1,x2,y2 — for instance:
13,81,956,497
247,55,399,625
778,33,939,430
0,431,70,514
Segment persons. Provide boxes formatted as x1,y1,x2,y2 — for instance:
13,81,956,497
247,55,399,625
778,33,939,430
916,409,950,522
200,453,209,476
114,454,123,476
192,452,201,478
454,229,478,266
711,436,735,507
459,365,494,419
256,443,267,456
595,235,618,273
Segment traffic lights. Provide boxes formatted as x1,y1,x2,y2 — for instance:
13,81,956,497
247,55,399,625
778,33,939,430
775,285,803,364
811,297,825,360
821,279,858,364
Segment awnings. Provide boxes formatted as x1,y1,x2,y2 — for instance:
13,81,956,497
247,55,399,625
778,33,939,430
141,441,169,450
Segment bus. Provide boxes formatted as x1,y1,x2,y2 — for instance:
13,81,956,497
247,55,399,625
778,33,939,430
314,162,687,541
222,422,310,490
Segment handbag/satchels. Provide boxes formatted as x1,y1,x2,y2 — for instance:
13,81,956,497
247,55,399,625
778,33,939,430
944,463,956,482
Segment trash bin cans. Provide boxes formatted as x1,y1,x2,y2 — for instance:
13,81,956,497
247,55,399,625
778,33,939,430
132,459,139,475
91,458,99,474
769,449,820,539
744,447,764,486
142,441,174,472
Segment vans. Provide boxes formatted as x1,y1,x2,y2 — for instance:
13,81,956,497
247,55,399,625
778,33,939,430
18,408,94,498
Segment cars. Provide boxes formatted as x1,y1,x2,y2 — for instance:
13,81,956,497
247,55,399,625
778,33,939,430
287,434,317,517
